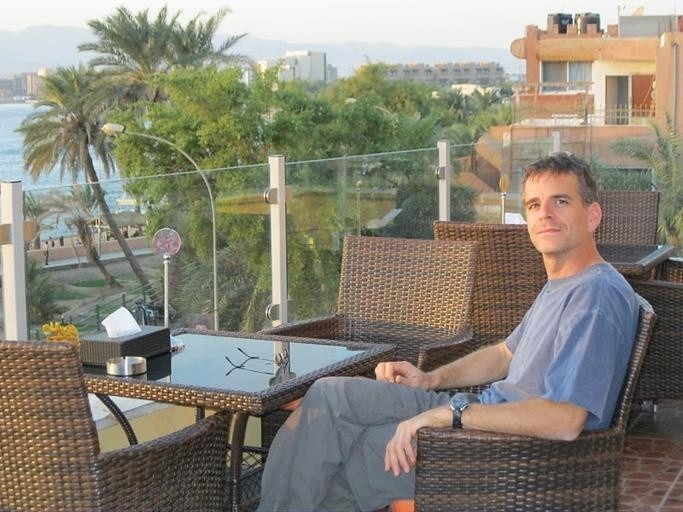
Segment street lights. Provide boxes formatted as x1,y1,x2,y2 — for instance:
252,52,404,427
100,122,220,332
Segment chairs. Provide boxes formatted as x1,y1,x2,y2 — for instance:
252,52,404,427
388,293,658,510
258,236,480,467
0,341,269,511
432,220,547,351
626,258,682,402
594,190,661,244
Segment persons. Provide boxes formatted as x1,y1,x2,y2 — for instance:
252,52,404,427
256,150,640,510
42,241,49,267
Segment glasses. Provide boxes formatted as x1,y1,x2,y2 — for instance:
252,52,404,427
225,347,289,375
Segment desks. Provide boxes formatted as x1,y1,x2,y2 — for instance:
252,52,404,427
83,327,398,512
595,243,672,279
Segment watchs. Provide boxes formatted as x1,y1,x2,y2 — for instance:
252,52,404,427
449,394,469,429
